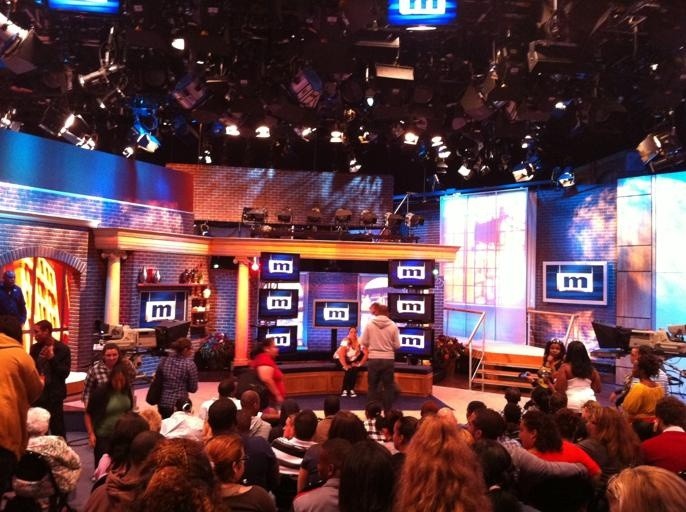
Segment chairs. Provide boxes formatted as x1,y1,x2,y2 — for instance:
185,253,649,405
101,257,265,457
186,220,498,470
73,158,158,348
21,450,77,511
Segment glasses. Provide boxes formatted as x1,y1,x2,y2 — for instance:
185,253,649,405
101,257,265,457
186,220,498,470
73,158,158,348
236,455,249,462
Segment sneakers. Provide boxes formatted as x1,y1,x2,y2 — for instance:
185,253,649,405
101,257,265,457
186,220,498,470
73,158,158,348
349,390,357,398
341,389,347,397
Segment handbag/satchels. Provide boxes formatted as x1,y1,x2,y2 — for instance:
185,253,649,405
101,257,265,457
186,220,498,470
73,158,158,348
237,368,269,410
146,367,161,405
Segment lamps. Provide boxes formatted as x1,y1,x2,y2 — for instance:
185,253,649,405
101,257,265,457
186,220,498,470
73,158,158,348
406,212,424,228
250,209,270,222
307,209,322,222
386,212,403,230
337,208,353,223
276,209,296,221
359,210,377,223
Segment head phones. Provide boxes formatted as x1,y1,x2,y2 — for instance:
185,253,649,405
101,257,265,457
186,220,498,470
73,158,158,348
182,399,191,410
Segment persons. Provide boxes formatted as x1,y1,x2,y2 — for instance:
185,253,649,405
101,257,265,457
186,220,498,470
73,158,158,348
158,394,205,441
84,368,134,456
545,340,602,411
146,337,198,415
85,433,272,511
0,313,46,492
24,318,71,438
0,269,28,344
527,339,565,392
362,303,401,410
273,399,461,511
614,346,672,417
84,345,136,394
462,390,686,511
250,337,288,406
206,378,272,438
16,404,83,510
334,327,368,399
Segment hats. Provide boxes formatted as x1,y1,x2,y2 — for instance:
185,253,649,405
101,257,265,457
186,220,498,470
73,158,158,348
261,407,281,421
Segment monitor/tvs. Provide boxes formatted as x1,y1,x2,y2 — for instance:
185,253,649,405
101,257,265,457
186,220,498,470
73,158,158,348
387,258,434,288
387,293,434,324
139,290,186,328
261,252,300,283
543,261,608,305
258,325,297,353
313,299,361,328
398,326,435,360
258,288,298,320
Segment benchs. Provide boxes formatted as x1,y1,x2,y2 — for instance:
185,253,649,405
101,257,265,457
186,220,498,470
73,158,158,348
273,361,434,400
